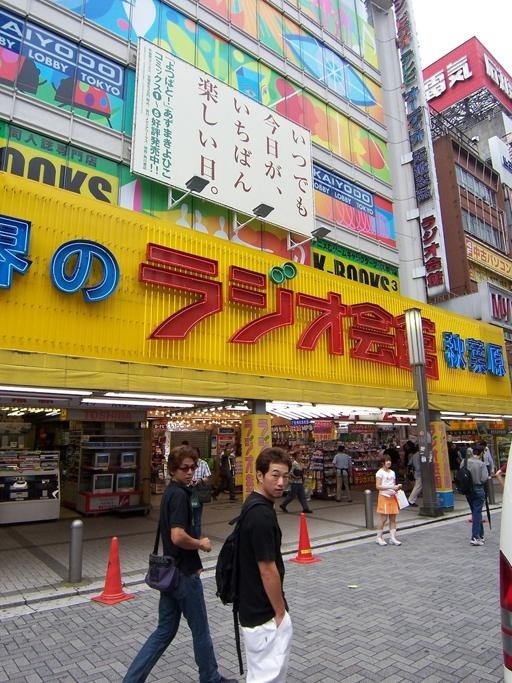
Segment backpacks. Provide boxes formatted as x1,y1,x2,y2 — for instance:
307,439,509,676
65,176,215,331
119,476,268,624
215,498,273,605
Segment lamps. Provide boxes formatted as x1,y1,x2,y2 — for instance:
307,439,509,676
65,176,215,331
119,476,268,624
285,226,330,253
166,175,210,211
232,202,274,235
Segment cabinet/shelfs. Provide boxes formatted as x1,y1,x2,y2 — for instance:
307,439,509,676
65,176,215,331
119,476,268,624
76,432,145,516
271,417,510,499
1,449,61,525
211,424,236,482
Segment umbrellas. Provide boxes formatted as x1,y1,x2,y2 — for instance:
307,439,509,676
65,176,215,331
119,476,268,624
483,480,492,530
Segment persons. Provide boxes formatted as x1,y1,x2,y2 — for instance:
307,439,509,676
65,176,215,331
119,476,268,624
150,428,509,516
187,448,213,539
216,446,293,682
123,444,238,682
459,443,490,546
375,453,403,546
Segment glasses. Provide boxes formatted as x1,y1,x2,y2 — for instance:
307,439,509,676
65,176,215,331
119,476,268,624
178,464,198,471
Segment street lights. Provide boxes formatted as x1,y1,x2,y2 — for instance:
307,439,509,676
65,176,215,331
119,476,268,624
403,306,445,519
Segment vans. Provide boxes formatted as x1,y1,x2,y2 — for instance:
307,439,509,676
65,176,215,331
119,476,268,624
496,440,512,681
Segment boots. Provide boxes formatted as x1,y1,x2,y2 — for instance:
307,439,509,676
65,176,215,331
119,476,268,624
389,530,402,545
376,530,387,545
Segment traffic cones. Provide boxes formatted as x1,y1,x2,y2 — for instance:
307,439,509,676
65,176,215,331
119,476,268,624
286,511,327,566
94,535,137,605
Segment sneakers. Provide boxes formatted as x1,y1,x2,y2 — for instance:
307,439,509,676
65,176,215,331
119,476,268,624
303,509,312,512
230,497,237,500
408,503,419,506
217,676,238,682
470,537,485,545
280,504,288,512
479,535,485,541
211,493,217,500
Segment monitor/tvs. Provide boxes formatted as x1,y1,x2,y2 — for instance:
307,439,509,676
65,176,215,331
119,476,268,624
117,452,137,468
90,473,114,494
116,473,135,493
91,453,111,466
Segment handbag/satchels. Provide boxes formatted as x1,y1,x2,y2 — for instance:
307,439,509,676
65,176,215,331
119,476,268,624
145,552,179,591
455,458,473,494
407,465,415,480
291,469,303,477
190,486,201,507
199,489,211,502
395,488,410,509
